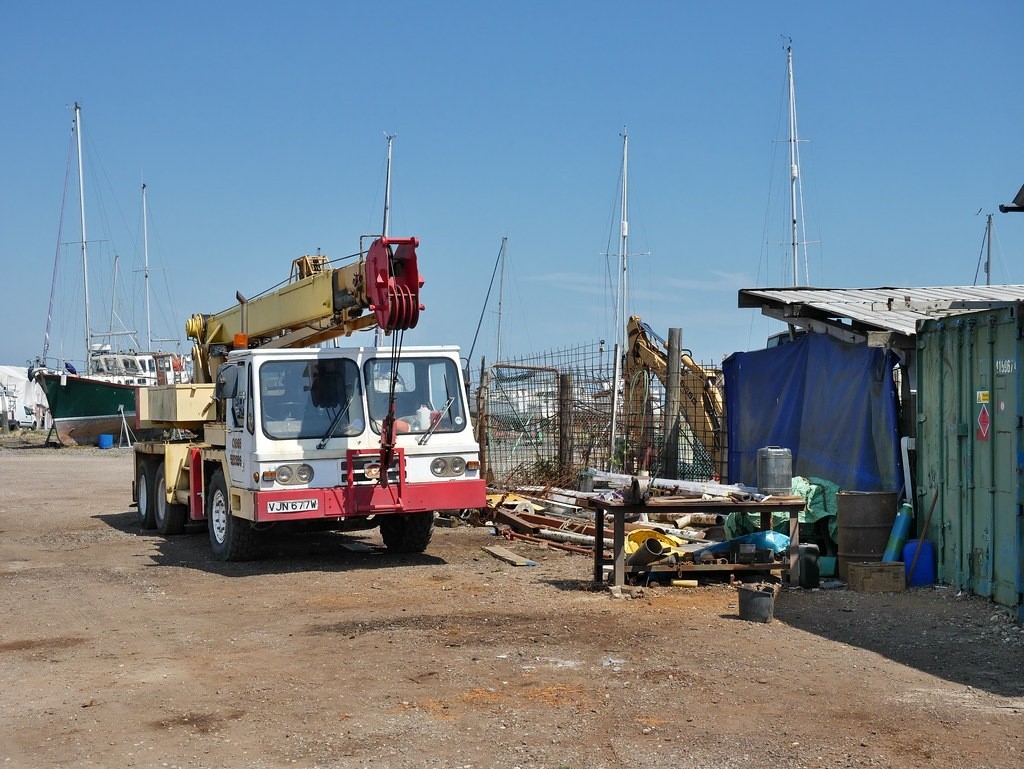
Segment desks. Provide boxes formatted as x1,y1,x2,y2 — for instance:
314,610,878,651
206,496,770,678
586,493,807,592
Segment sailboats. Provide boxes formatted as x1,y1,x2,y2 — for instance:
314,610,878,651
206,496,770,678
27,103,202,448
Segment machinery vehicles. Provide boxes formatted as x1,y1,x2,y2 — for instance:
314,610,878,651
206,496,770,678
127,232,488,563
622,314,729,483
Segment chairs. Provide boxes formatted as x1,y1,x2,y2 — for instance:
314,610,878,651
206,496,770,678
381,395,437,420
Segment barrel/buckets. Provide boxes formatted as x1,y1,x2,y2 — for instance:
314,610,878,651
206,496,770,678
902,539,936,586
736,585,774,623
756,446,792,496
781,542,820,590
836,491,898,583
99,434,114,448
